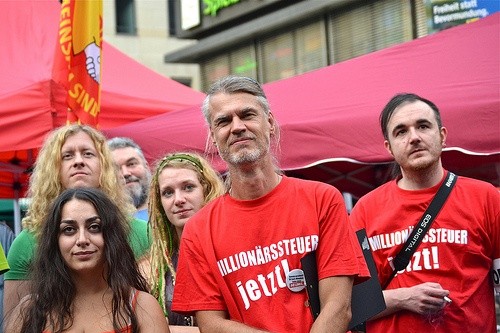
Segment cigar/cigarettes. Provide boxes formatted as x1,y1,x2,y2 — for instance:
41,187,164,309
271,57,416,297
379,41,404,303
443,296,452,304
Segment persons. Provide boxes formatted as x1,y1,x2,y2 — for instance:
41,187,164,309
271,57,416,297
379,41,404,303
349,93,500,333
146,153,224,333
171,75,371,333
0,125,171,333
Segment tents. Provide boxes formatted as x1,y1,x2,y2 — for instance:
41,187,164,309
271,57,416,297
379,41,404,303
97,12,500,200
0,0,208,237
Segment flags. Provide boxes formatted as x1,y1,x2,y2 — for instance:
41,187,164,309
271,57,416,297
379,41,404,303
51,0,105,131
